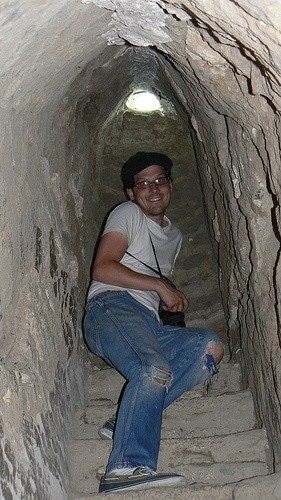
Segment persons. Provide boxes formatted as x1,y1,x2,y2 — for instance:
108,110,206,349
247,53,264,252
84,152,225,493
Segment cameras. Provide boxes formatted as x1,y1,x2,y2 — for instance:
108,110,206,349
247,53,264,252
159,303,185,327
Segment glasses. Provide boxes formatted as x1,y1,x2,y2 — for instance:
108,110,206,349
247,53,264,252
132,177,170,189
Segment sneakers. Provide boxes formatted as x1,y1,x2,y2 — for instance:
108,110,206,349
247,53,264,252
97,417,117,440
98,466,185,495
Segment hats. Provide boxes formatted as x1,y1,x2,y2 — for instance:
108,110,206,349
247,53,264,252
121,151,173,186
